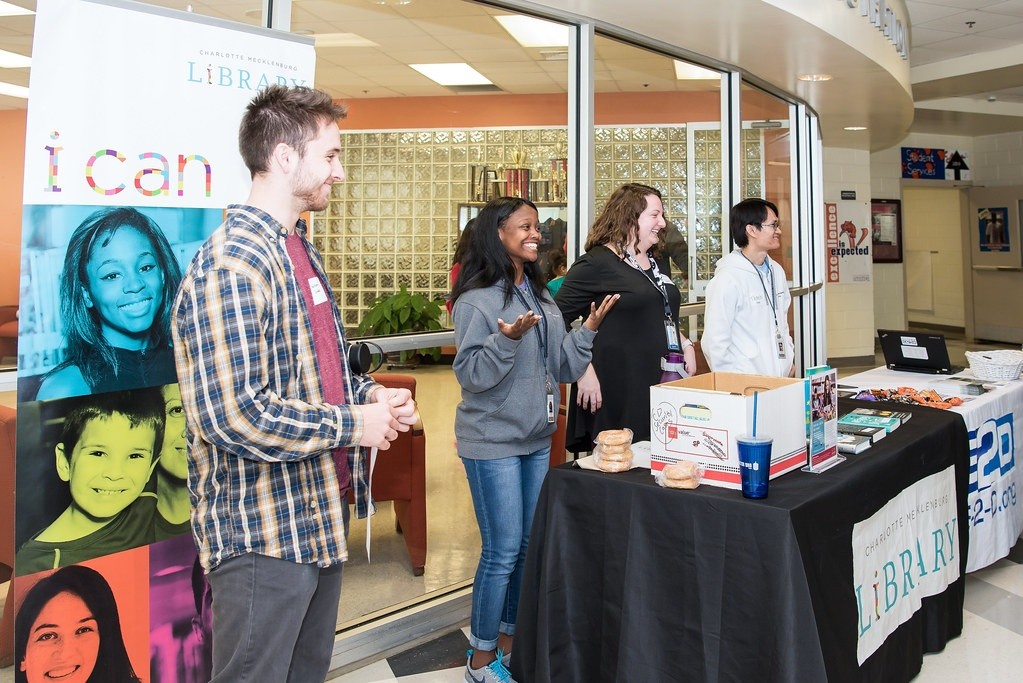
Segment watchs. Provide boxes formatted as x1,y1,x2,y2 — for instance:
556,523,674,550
682,338,695,350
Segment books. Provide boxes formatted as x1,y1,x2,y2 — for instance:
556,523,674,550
851,408,912,424
838,424,886,443
836,434,872,454
838,413,901,433
806,364,830,438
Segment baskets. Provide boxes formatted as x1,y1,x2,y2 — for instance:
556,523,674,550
965,348,1023,381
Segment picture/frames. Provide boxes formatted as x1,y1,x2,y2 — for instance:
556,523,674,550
871,198,903,264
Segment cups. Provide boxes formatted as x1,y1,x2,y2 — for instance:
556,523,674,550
735,437,773,500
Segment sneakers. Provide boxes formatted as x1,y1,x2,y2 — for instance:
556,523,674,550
463,649,519,683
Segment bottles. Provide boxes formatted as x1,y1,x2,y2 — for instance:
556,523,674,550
346,342,382,376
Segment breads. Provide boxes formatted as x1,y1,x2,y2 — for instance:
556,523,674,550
596,430,632,473
661,460,701,489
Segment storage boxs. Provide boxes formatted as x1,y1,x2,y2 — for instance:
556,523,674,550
649,371,807,491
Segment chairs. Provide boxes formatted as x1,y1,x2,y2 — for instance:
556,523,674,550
348,374,428,576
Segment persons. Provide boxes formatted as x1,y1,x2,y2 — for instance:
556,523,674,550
446,195,621,683
549,401,552,412
168,85,421,683
779,343,783,351
700,197,795,379
555,183,695,453
654,218,688,281
812,375,834,421
13,207,215,683
985,212,1004,250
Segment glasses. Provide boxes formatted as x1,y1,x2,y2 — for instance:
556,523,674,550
753,221,781,230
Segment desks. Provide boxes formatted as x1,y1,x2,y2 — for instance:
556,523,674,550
509,396,969,683
837,364,1023,573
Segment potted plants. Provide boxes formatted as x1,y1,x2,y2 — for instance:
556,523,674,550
352,282,443,370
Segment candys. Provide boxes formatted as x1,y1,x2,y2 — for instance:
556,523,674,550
854,388,963,410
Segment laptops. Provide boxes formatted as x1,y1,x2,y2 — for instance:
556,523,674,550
877,329,966,375
